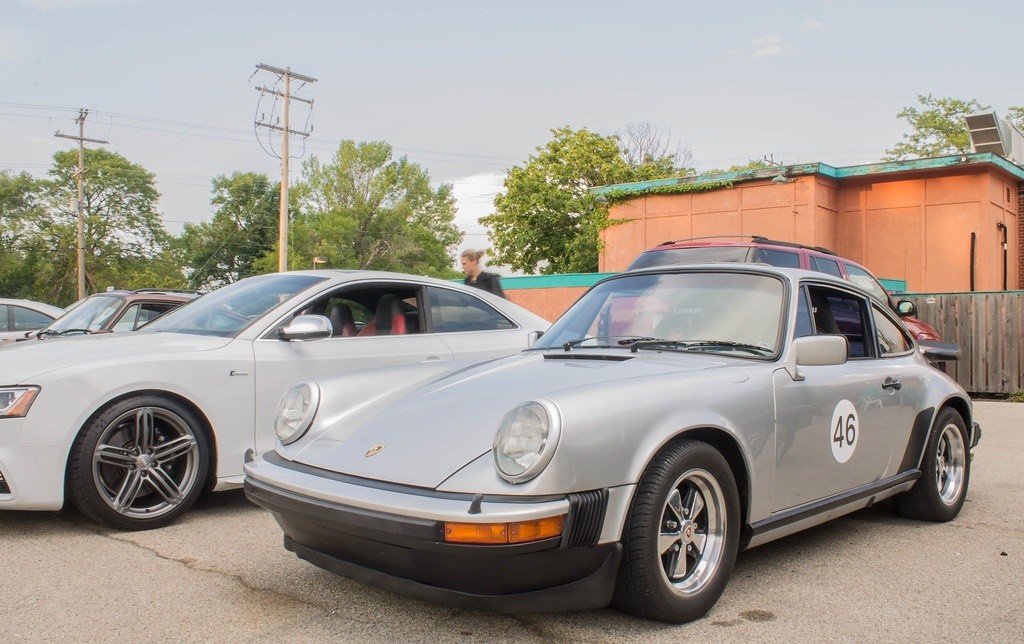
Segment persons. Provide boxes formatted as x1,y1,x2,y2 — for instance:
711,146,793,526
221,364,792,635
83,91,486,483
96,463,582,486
461,248,505,299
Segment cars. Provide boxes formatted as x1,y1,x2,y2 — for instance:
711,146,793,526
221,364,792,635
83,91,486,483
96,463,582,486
0,287,256,341
0,266,605,533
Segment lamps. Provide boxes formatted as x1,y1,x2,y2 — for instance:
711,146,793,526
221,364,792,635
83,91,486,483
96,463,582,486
772,170,786,185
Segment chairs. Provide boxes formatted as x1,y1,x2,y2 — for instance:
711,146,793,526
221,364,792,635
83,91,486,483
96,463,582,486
810,291,841,335
356,294,406,336
330,303,357,338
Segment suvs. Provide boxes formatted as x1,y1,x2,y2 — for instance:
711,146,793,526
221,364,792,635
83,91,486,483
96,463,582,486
596,233,950,376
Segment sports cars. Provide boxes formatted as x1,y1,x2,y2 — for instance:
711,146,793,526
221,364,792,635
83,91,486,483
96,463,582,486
242,262,984,625
